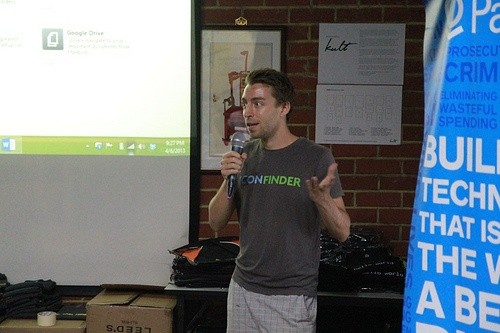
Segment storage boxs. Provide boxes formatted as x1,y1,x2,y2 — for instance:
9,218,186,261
0,318,85,333
86,284,177,333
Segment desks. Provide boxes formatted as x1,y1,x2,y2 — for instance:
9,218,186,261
164,283,403,333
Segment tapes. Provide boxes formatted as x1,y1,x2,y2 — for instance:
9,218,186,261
37,311,57,326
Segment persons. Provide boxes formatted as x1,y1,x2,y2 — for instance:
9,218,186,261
207,69,350,333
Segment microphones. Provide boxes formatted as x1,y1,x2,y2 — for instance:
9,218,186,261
229,132,246,197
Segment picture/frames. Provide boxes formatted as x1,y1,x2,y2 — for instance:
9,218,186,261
197,26,287,175
